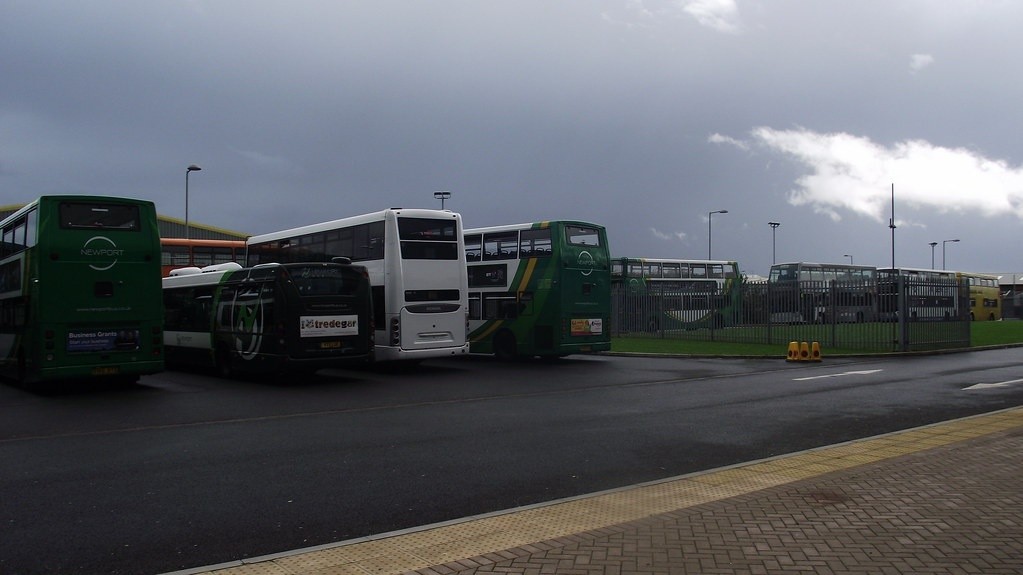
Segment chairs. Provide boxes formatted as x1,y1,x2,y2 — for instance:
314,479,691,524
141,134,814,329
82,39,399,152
466,247,554,261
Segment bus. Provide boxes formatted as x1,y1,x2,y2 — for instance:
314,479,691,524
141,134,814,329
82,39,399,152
162,263,376,384
421,221,613,364
243,207,471,377
768,262,878,324
0,194,163,396
955,272,1002,322
876,267,958,321
609,257,744,334
151,237,312,277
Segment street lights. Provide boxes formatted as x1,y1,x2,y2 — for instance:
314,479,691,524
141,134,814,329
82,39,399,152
928,241,938,270
844,254,852,265
708,210,728,261
185,165,202,241
433,192,451,234
943,238,960,270
768,220,781,282
